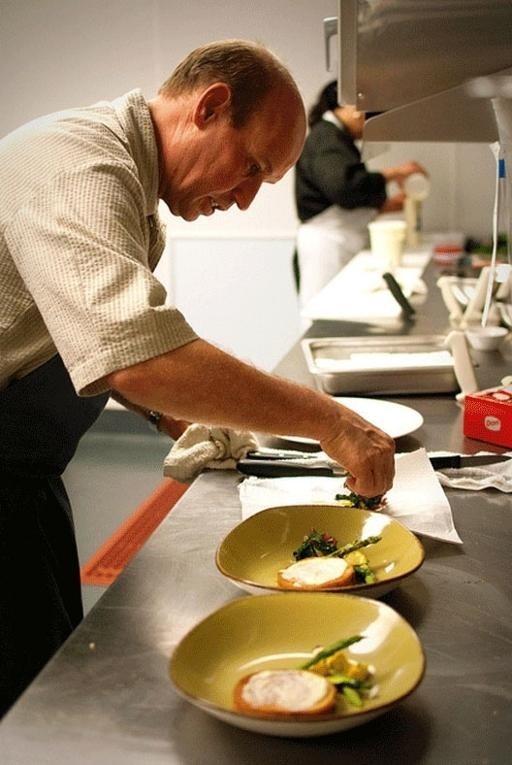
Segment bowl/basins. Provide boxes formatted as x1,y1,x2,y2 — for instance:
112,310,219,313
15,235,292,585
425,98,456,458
466,325,509,351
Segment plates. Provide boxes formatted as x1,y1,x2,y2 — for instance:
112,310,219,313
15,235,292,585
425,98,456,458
270,396,424,445
165,591,428,737
214,504,427,601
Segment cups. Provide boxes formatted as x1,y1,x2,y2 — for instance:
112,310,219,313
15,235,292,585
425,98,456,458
368,219,407,277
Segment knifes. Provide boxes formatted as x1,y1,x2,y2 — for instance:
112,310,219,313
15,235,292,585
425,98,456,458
428,453,512,473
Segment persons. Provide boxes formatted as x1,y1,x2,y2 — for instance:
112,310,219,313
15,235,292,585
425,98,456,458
292,77,434,338
1,37,397,723
